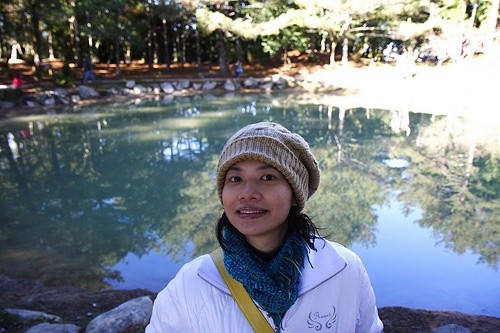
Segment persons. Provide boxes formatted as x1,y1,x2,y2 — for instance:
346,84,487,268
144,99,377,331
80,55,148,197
83,53,96,85
233,59,242,77
384,37,437,63
144,122,384,333
13,72,25,89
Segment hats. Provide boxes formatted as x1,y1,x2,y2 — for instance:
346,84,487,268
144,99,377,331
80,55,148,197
216,122,321,213
